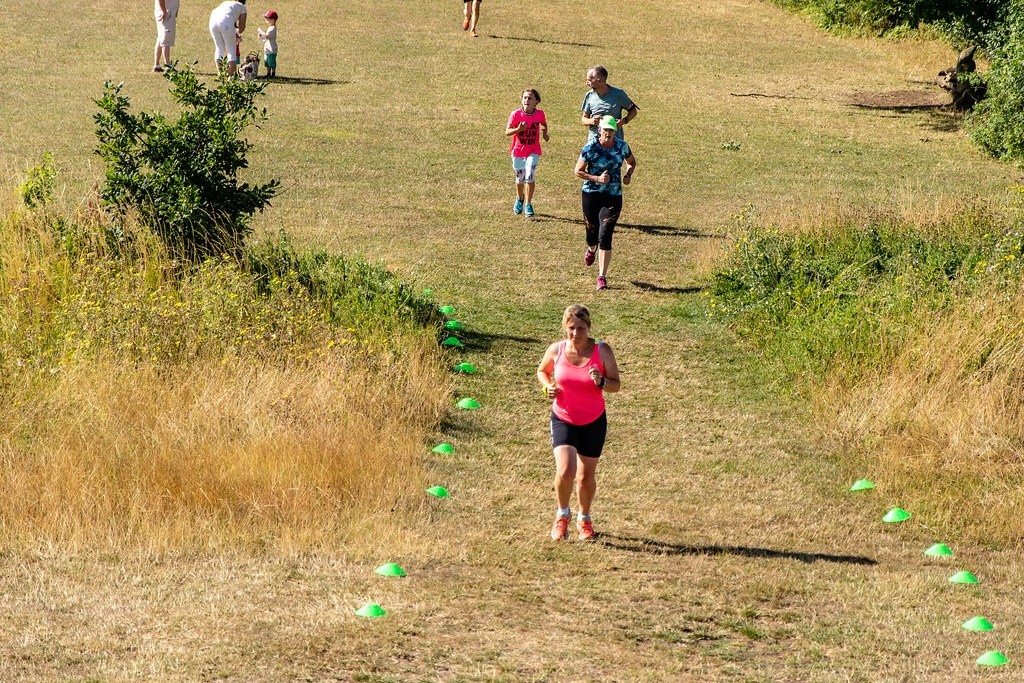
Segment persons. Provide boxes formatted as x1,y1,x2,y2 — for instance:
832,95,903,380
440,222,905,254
463,0,482,37
258,10,279,80
152,0,180,73
580,66,640,139
210,0,247,81
505,89,550,217
575,114,636,291
537,303,621,544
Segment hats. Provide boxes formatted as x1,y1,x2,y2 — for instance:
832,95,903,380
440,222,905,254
599,114,618,131
261,10,278,21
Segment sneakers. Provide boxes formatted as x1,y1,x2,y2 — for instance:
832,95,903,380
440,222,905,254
584,245,599,266
577,519,596,542
596,276,607,290
551,509,572,541
470,30,478,37
524,204,534,215
514,194,524,214
463,16,471,30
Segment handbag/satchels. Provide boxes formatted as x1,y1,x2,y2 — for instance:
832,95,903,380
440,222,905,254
239,51,259,80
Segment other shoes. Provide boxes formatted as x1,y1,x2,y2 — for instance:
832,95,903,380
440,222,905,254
154,67,165,72
263,75,270,79
269,76,275,79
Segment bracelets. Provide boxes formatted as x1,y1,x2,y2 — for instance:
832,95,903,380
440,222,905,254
597,376,607,390
623,117,629,124
542,385,548,396
